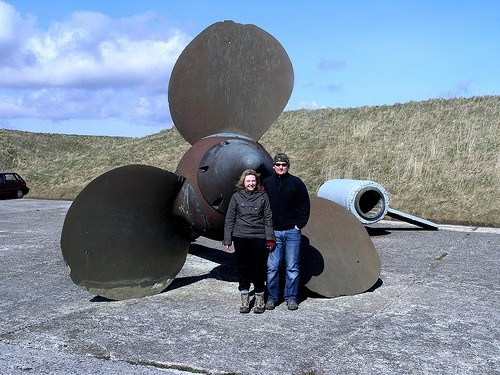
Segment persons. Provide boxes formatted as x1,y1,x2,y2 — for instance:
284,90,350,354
262,153,310,310
222,169,276,313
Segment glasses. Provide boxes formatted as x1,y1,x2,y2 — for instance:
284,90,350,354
274,164,287,166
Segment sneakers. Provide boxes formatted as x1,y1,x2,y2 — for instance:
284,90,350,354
254,295,265,312
266,301,274,309
240,294,249,312
288,301,298,309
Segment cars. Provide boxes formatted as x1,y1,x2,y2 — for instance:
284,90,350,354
0,170,29,200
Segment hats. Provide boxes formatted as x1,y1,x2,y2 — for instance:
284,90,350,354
272,153,290,167
